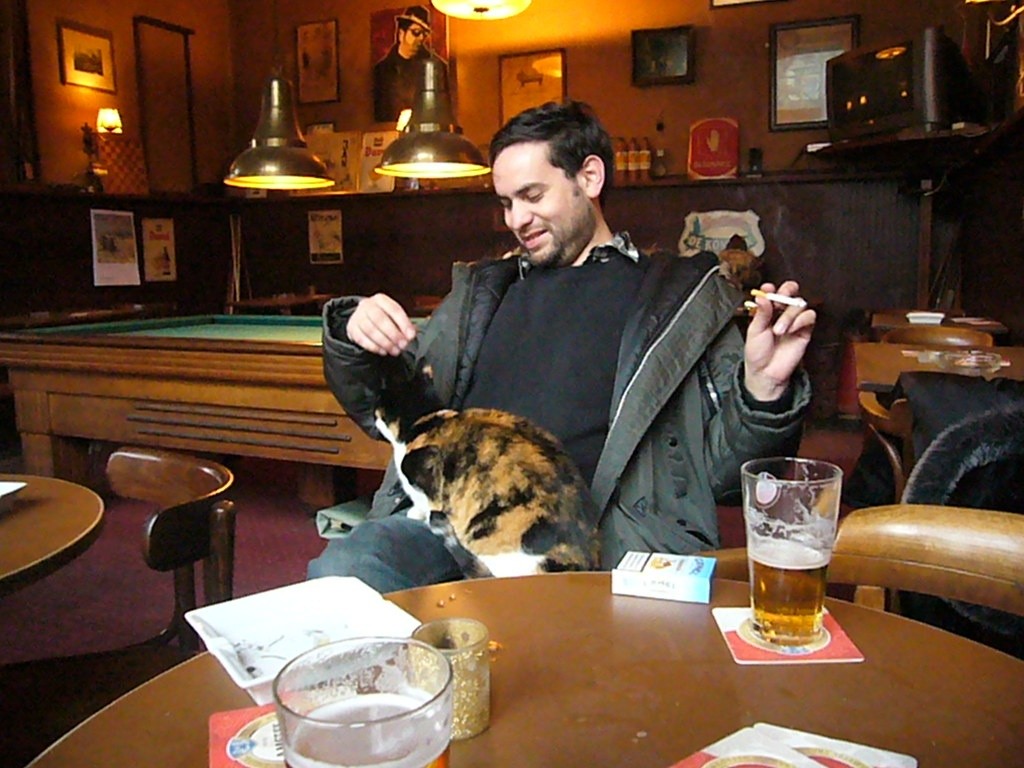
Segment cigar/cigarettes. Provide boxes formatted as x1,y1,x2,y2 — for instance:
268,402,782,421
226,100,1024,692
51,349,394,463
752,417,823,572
751,289,806,309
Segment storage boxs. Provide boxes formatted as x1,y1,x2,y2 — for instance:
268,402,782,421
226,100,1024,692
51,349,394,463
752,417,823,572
611,551,717,603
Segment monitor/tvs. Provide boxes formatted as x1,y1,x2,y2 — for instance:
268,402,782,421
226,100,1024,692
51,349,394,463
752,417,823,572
826,25,975,143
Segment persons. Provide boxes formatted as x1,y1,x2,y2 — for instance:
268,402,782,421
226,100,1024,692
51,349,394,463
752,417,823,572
307,97,816,594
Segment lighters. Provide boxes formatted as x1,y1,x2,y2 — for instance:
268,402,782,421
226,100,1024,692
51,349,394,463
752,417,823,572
744,301,785,325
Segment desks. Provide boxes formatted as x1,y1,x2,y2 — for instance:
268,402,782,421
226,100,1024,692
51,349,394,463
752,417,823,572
813,122,998,311
854,345,1024,393
25,572,1024,768
0,258,192,326
869,307,1009,344
0,473,104,600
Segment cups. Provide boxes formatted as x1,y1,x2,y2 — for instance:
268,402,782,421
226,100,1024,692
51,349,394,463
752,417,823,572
412,617,491,742
740,457,844,654
271,635,455,768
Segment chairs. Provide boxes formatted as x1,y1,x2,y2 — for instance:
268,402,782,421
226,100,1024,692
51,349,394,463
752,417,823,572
858,326,999,504
0,447,239,768
701,504,1024,617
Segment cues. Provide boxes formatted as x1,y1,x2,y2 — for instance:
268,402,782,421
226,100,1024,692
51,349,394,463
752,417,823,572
225,212,253,315
924,208,971,309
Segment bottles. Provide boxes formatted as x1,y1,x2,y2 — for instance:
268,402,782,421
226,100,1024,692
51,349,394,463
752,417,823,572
615,137,651,180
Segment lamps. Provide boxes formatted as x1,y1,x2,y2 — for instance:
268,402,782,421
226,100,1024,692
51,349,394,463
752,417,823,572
374,56,492,180
222,78,334,190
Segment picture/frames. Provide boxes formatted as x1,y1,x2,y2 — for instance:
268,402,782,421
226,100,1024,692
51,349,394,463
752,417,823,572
133,15,197,200
498,48,568,129
294,18,342,104
768,14,861,131
629,23,697,86
55,16,120,95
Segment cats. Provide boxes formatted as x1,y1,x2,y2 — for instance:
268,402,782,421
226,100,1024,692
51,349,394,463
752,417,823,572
371,362,607,579
